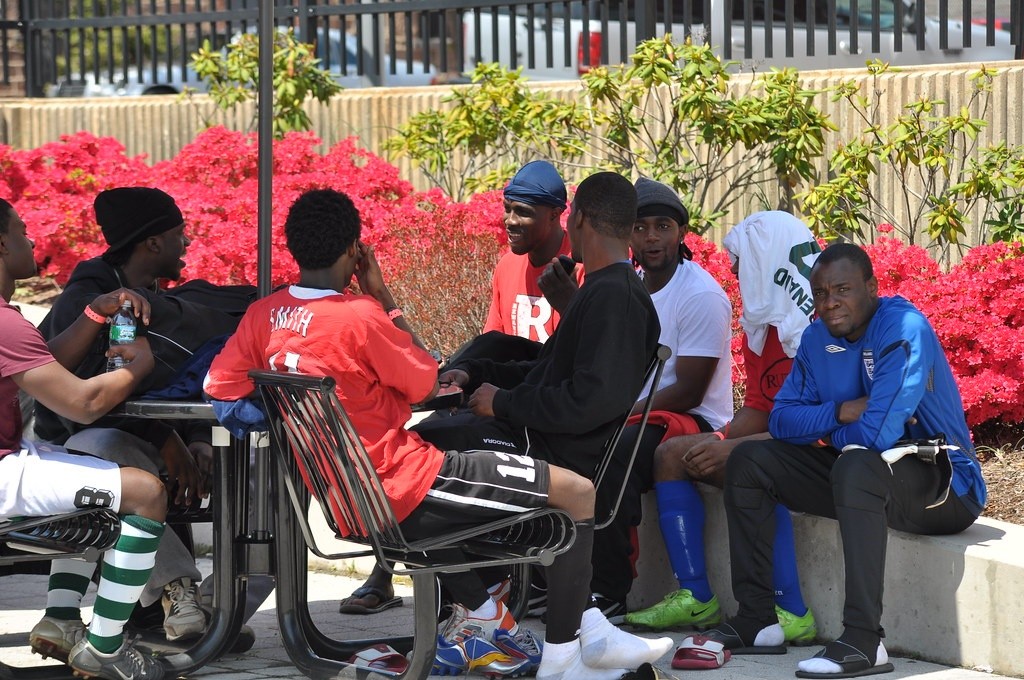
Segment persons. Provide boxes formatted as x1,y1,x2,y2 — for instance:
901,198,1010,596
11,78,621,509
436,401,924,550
34,187,256,654
0,197,169,680
623,210,827,645
694,243,987,680
201,188,673,680
341,158,736,629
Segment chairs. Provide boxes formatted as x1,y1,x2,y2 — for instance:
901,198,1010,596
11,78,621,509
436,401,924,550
0,475,215,680
247,343,673,679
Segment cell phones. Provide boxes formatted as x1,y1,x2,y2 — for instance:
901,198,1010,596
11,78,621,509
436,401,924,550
552,254,575,276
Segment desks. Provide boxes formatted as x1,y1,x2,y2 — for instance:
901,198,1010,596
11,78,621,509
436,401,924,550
34,383,462,680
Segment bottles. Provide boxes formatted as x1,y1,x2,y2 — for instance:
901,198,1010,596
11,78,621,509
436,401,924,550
106,300,136,372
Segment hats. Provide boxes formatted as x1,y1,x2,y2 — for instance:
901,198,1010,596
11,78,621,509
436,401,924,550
636,178,690,226
505,161,568,207
95,187,182,252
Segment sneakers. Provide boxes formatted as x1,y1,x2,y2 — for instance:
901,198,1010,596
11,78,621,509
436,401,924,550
68,637,166,680
431,634,530,679
489,627,544,669
443,604,517,645
162,578,208,642
30,619,86,662
775,606,816,647
489,579,511,602
625,589,722,631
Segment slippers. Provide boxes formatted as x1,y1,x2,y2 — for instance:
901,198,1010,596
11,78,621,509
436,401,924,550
340,587,403,614
699,617,786,653
623,662,677,680
671,636,731,669
345,642,408,673
796,642,893,678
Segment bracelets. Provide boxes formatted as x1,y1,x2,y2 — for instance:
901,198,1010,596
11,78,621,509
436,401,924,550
712,421,730,442
84,305,106,324
386,306,402,320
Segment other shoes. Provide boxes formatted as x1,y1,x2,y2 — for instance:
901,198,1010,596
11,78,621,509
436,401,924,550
528,581,550,618
540,590,624,623
